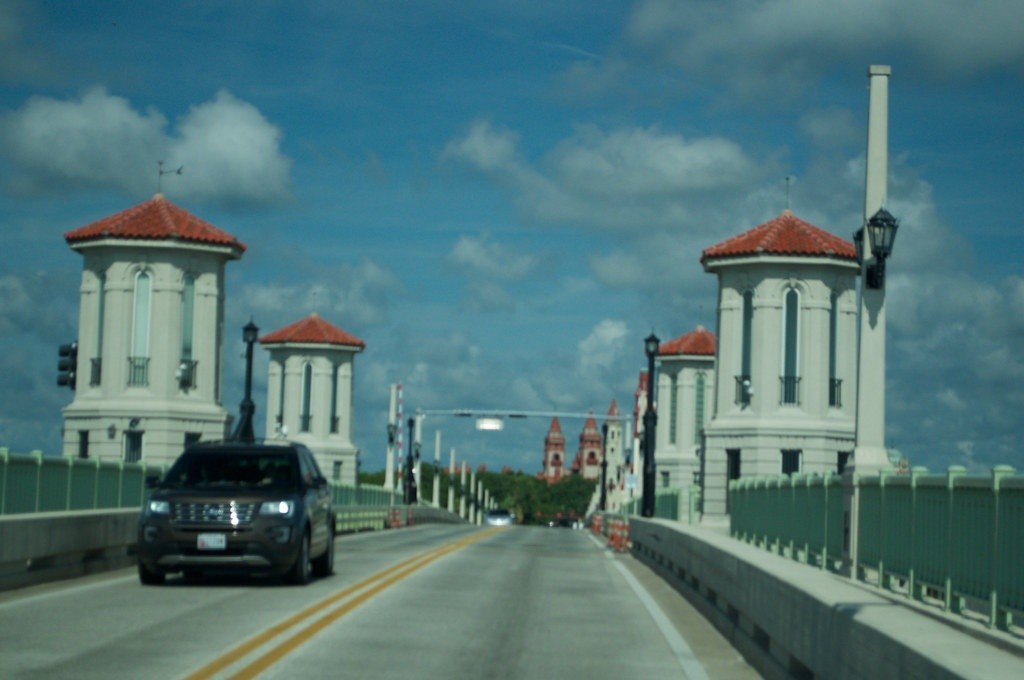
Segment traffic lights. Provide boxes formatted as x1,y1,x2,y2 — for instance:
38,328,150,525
57,340,78,390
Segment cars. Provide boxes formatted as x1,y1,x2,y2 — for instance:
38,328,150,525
485,508,517,526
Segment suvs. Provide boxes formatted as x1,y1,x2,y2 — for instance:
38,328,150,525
136,437,337,585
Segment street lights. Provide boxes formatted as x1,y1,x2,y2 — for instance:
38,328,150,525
641,330,663,518
384,423,398,490
842,203,900,470
230,315,260,443
402,417,415,505
599,421,608,511
